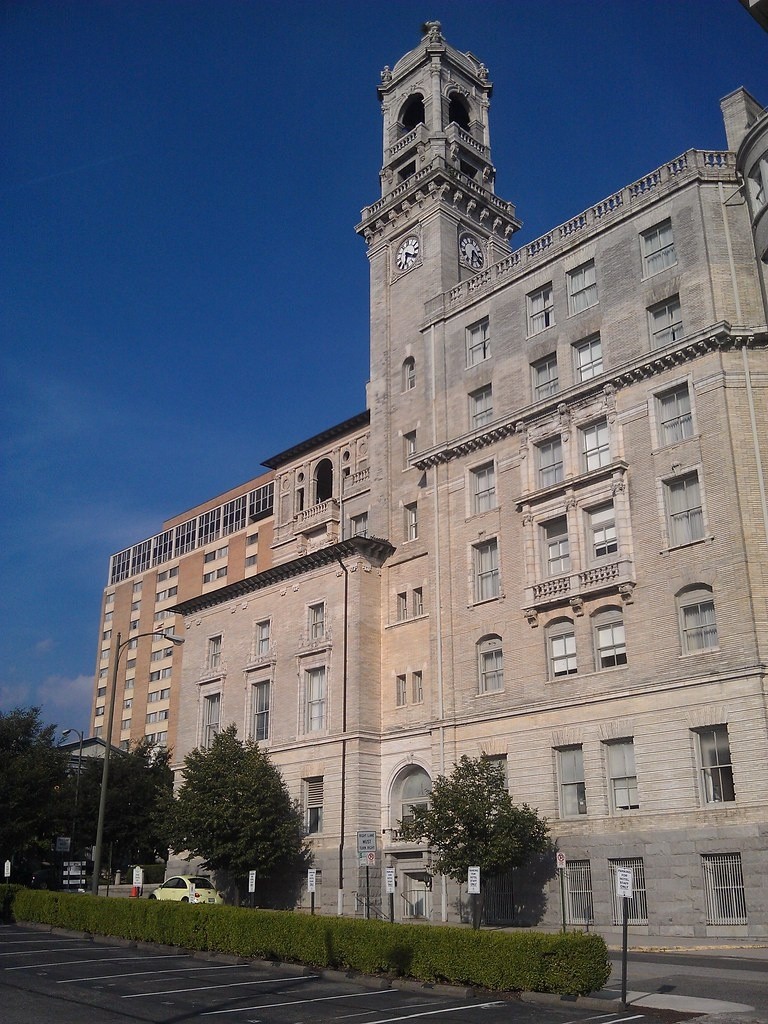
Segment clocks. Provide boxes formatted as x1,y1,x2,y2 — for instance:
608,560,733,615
395,236,420,270
460,237,484,268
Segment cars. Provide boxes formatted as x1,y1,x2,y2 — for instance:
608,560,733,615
149,874,225,906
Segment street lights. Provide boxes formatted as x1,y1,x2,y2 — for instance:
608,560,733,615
62,728,85,892
91,632,186,896
